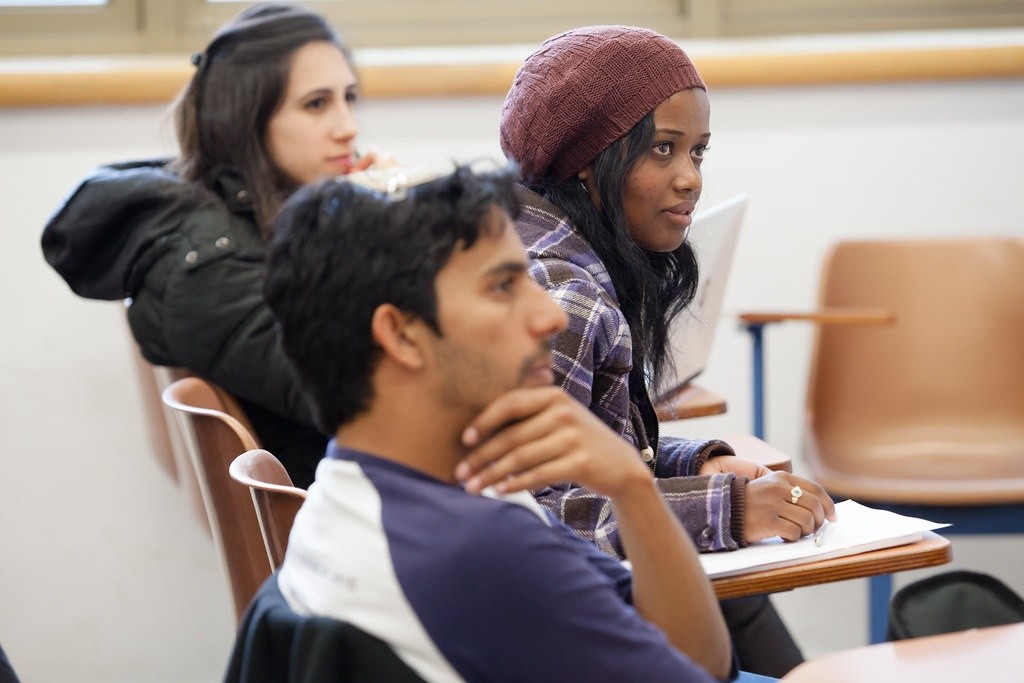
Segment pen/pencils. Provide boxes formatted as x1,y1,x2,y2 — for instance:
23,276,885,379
814,520,833,546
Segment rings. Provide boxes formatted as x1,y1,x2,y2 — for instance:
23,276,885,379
790,485,802,503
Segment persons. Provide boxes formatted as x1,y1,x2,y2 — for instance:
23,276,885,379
223,165,731,683
129,0,397,484
499,25,840,560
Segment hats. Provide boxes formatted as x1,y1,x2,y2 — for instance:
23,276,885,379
499,26,707,189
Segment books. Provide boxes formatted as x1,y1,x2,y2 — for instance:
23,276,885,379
619,518,954,580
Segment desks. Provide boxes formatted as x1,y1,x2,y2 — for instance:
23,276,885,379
779,618,1022,681
622,496,952,641
736,304,900,437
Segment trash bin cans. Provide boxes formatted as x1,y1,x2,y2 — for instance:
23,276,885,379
884,569,1024,642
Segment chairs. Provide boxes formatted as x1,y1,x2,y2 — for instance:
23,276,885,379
117,300,347,638
801,228,1024,642
